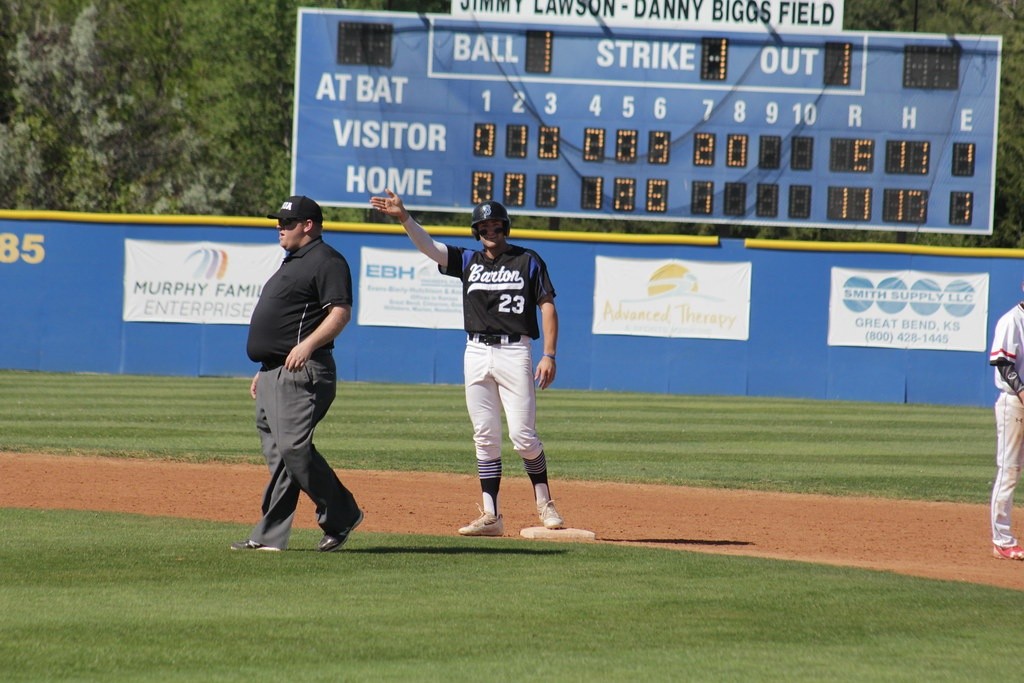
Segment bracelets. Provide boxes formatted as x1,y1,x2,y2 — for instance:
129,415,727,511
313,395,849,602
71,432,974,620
542,353,556,358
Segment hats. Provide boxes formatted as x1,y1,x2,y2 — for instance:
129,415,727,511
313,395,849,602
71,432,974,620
267,196,323,222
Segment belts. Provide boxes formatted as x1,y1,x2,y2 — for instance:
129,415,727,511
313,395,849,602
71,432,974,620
469,333,521,346
261,349,331,368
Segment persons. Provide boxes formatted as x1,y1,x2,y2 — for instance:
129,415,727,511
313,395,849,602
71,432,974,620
988,279,1024,559
230,197,365,554
370,189,564,535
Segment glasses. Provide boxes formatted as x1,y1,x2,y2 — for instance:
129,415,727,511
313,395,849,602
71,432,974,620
278,218,304,225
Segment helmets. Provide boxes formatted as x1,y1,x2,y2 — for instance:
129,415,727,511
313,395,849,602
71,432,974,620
471,201,510,241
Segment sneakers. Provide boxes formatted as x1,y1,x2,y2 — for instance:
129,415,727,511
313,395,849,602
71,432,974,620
318,509,364,552
537,500,563,529
994,544,1024,559
231,540,281,551
459,503,504,536
